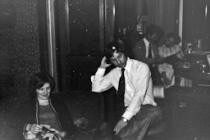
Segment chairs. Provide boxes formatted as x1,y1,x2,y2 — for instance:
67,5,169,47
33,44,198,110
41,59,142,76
146,65,176,140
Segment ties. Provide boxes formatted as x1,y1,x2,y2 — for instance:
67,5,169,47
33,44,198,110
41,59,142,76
117,68,125,121
148,43,153,58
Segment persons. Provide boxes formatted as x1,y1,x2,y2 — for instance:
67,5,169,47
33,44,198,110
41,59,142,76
135,27,169,101
90,41,162,140
153,33,183,103
9,73,75,140
